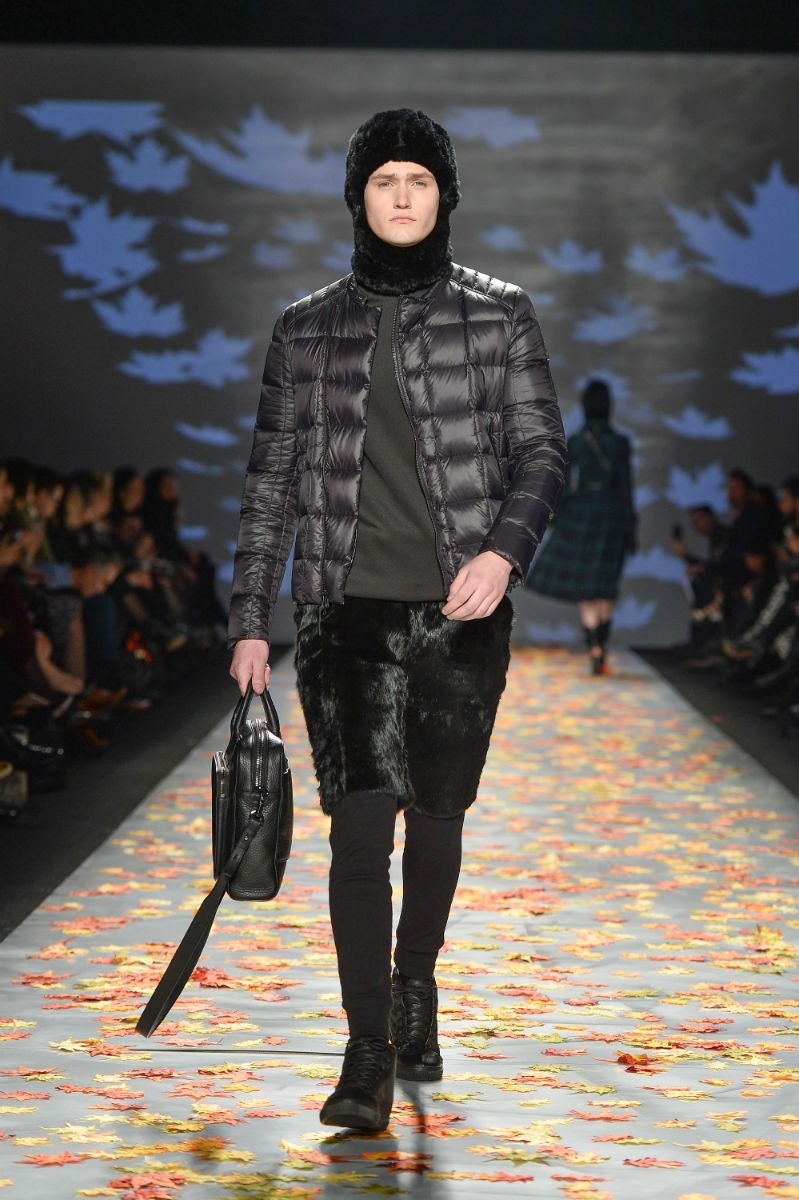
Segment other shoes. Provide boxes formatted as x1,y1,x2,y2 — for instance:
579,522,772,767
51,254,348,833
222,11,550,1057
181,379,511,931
583,628,610,674
683,629,799,731
0,620,218,798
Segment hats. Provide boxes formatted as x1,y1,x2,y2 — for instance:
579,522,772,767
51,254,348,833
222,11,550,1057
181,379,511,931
345,110,458,296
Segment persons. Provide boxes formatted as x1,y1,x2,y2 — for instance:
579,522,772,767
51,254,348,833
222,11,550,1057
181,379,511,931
523,380,638,675
0,458,229,805
229,109,568,1129
671,468,799,719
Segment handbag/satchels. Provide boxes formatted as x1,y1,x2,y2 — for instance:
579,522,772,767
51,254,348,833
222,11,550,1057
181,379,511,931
210,676,295,903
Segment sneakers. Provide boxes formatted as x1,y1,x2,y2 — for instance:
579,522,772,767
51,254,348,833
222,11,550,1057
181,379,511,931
390,968,443,1081
320,1037,395,1133
